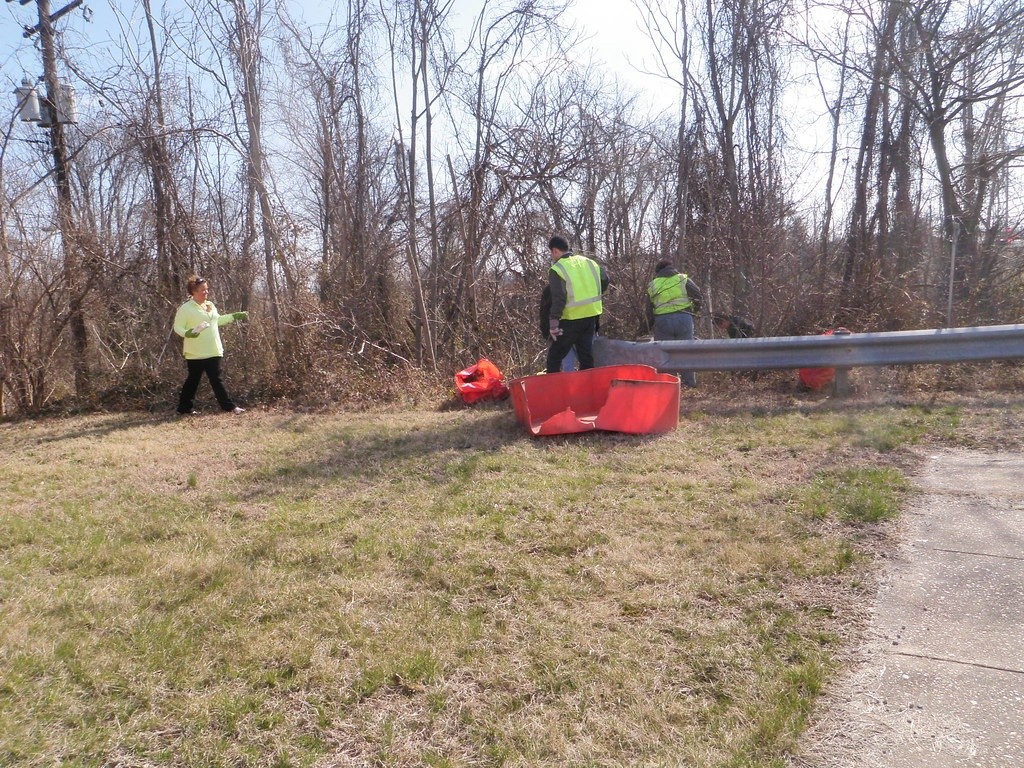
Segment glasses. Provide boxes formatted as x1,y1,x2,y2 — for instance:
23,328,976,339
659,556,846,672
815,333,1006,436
196,278,205,285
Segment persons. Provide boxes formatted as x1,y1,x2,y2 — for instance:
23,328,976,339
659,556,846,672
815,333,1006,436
646,260,702,388
540,235,610,374
174,275,249,414
713,313,762,339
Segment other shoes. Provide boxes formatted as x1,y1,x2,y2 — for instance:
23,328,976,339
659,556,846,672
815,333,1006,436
233,407,246,415
191,411,202,417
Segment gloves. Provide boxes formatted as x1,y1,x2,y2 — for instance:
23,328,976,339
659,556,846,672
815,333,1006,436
185,328,200,338
549,328,564,340
234,312,248,320
